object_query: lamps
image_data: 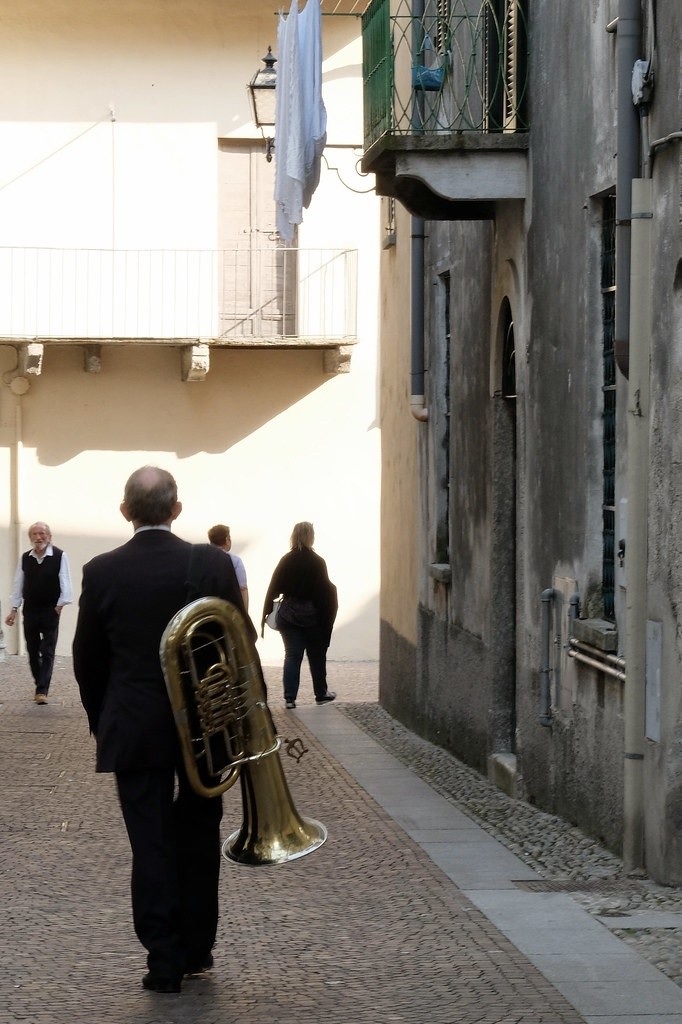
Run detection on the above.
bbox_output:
[248,46,281,165]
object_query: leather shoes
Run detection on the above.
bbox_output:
[189,955,214,975]
[140,973,182,993]
[34,694,48,704]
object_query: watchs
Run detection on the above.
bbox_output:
[11,608,18,614]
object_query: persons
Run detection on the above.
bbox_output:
[207,524,250,618]
[4,520,72,705]
[72,464,266,994]
[259,520,338,709]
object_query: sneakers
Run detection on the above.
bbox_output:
[285,701,295,708]
[316,692,336,704]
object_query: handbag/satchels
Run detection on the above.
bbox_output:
[266,600,283,631]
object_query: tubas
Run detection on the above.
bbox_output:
[158,595,328,868]
